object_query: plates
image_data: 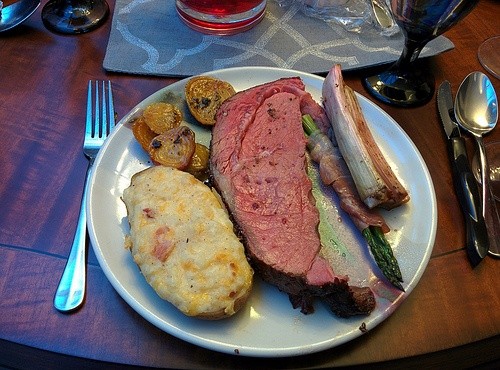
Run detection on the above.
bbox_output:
[87,68,440,357]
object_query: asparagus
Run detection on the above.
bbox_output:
[302,114,406,292]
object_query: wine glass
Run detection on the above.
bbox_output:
[363,0,481,108]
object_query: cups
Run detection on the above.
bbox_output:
[173,1,267,37]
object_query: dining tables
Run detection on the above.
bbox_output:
[0,0,498,369]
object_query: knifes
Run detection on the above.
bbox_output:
[432,81,490,269]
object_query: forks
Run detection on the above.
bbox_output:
[53,79,122,313]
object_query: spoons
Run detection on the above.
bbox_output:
[454,71,500,258]
